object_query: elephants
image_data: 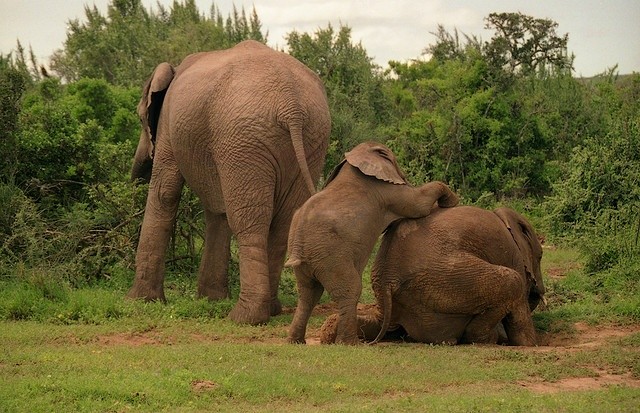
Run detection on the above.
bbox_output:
[282,139,462,346]
[318,204,548,347]
[128,37,333,327]
